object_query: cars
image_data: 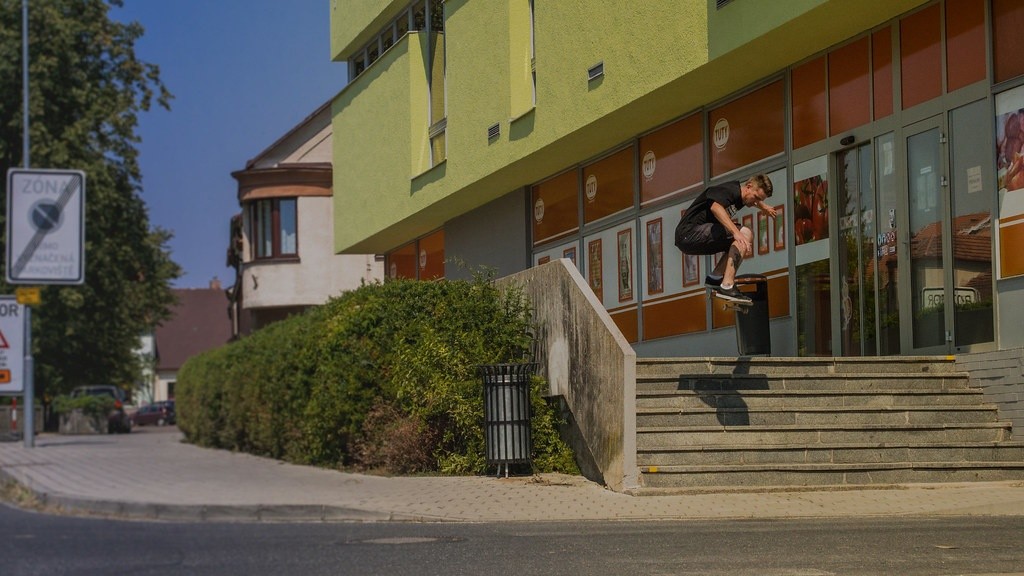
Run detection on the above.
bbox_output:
[69,384,132,434]
[127,400,177,428]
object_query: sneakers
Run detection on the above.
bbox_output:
[704,275,724,289]
[716,284,755,306]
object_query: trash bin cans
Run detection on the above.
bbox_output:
[474,362,540,478]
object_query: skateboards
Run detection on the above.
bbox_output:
[706,284,754,316]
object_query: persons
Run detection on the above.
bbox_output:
[674,173,777,303]
